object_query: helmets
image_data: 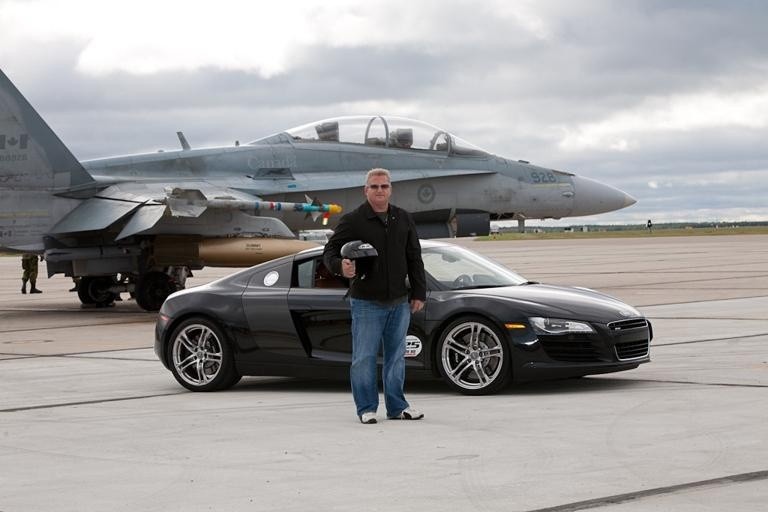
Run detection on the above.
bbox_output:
[340,241,379,278]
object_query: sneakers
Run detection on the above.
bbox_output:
[361,412,377,424]
[397,406,424,420]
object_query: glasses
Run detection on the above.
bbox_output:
[369,184,391,189]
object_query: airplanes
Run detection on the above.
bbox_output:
[1,66,642,315]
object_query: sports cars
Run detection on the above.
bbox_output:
[150,238,653,399]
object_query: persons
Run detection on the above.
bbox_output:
[322,168,427,423]
[21,252,44,293]
[69,278,81,291]
